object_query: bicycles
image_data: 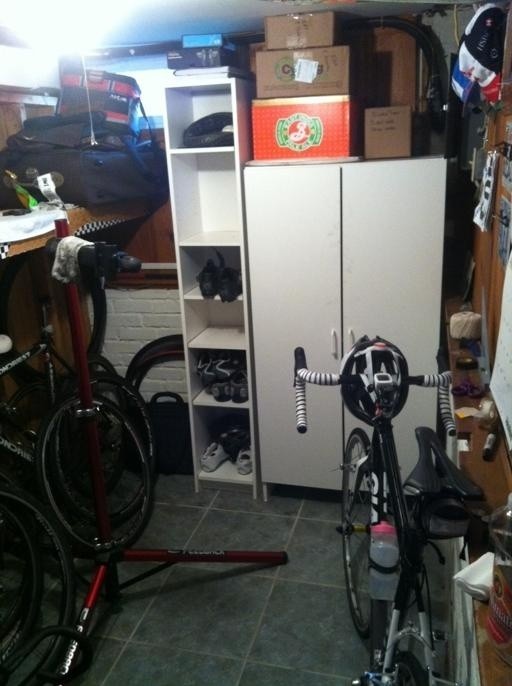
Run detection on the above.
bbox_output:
[293,334,486,686]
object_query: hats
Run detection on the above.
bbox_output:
[450,3,509,118]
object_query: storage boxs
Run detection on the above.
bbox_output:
[264,11,335,51]
[249,41,264,73]
[364,105,411,160]
[167,46,239,69]
[182,34,236,49]
[251,95,358,158]
[256,45,359,98]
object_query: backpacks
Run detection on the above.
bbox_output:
[3,109,167,203]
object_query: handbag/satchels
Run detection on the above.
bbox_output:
[125,390,193,476]
[55,66,144,137]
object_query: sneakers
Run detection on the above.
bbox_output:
[199,429,251,475]
[198,258,241,303]
[197,351,248,403]
[338,334,409,425]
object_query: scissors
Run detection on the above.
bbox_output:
[452,374,484,398]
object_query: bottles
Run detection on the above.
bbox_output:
[368,519,399,603]
[490,491,512,666]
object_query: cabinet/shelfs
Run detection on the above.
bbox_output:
[243,158,448,502]
[163,76,259,501]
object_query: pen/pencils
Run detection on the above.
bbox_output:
[482,422,500,462]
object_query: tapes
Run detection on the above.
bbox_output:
[456,357,478,370]
